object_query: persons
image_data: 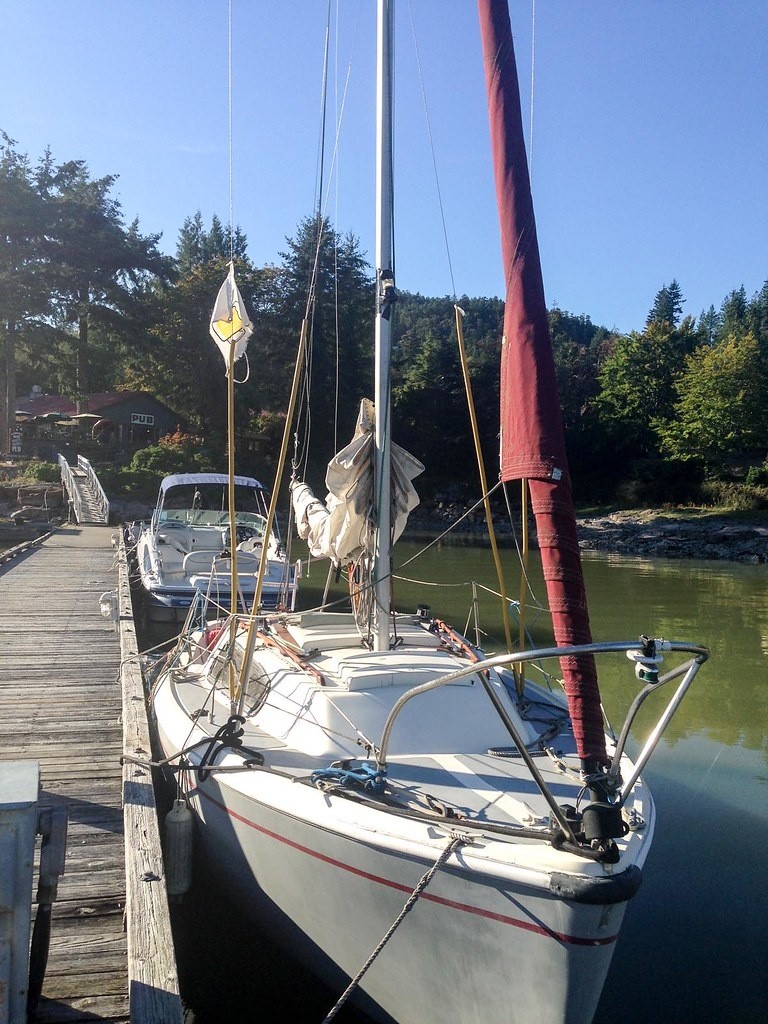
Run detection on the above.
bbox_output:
[194,491,202,509]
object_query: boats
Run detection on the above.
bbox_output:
[136,473,300,622]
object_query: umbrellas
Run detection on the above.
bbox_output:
[15,410,102,440]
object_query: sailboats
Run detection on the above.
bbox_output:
[149,0,709,1024]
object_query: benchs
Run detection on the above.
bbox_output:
[184,550,257,579]
